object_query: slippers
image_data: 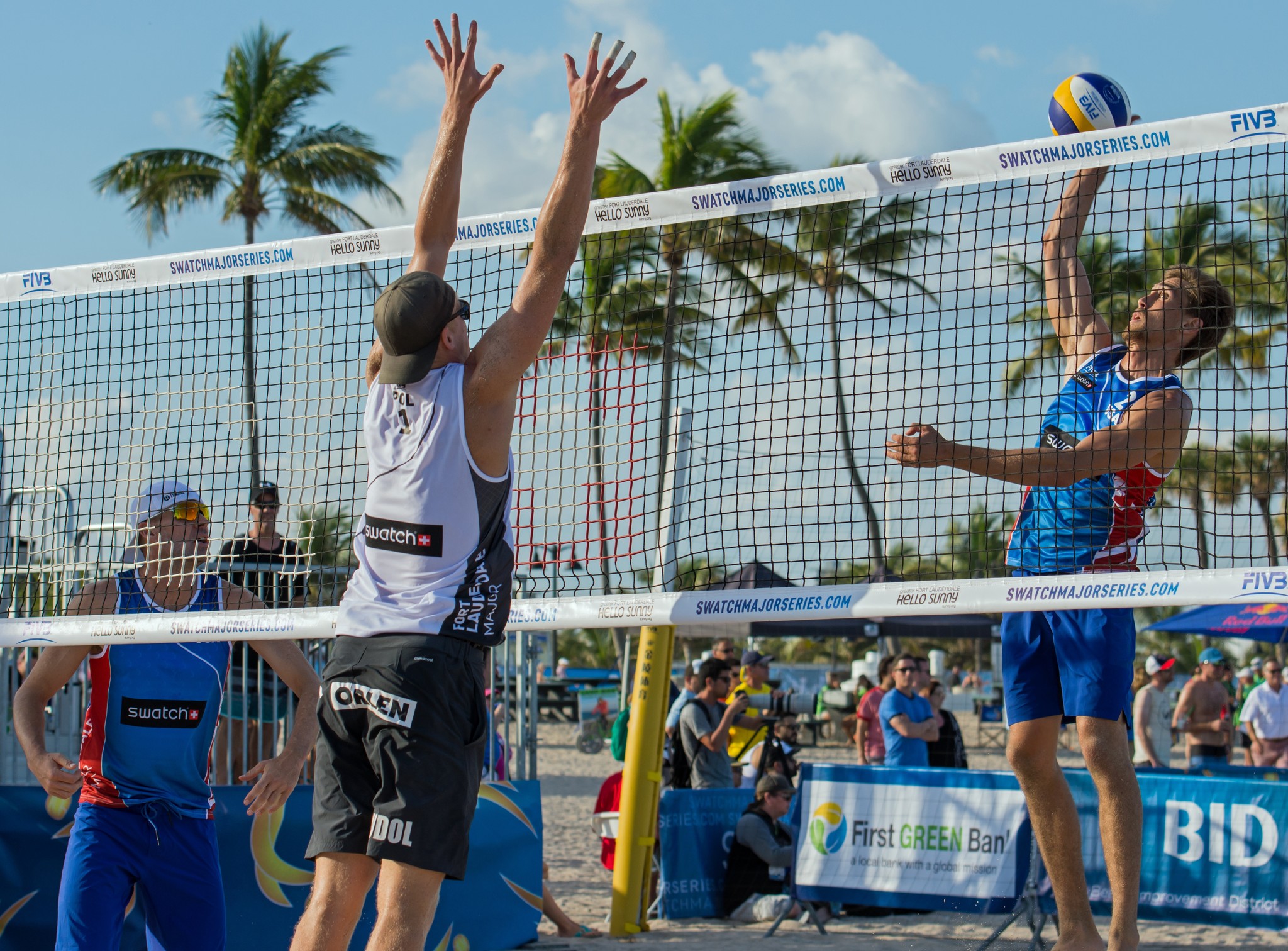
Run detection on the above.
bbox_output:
[574,925,604,938]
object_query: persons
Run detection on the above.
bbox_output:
[649,638,834,927]
[1171,646,1233,767]
[212,482,313,786]
[12,478,323,951]
[816,652,985,770]
[480,645,604,940]
[1131,652,1288,770]
[907,115,1235,951]
[288,12,647,951]
[8,648,41,701]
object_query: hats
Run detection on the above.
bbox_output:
[1199,647,1228,665]
[373,270,457,384]
[485,688,501,697]
[756,775,798,795]
[1250,656,1263,670]
[765,739,801,767]
[1282,666,1288,682]
[740,651,774,666]
[1145,653,1175,677]
[119,480,204,565]
[248,481,280,501]
[1235,666,1253,678]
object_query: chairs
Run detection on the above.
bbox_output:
[785,692,820,747]
[973,695,1006,750]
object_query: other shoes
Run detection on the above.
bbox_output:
[796,909,810,924]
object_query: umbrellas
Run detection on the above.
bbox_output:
[1141,603,1288,646]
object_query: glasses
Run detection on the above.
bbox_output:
[731,672,739,678]
[721,648,734,654]
[715,676,732,683]
[782,796,791,801]
[447,299,472,325]
[1268,669,1283,673]
[778,723,801,730]
[895,666,915,673]
[148,502,211,522]
[253,500,281,509]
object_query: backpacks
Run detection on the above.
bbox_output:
[610,706,632,762]
[669,698,725,790]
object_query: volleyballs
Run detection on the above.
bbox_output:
[1048,71,1132,136]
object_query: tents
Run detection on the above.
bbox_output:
[695,559,1001,642]
